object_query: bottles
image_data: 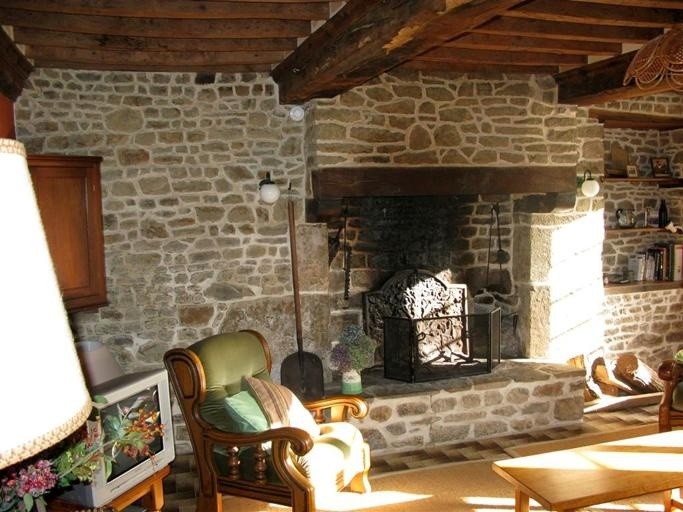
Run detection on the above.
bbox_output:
[657,198,667,228]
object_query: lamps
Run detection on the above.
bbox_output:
[0,136,93,467]
[577,170,599,199]
[254,169,290,206]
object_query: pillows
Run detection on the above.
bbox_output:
[243,375,320,437]
[223,391,270,450]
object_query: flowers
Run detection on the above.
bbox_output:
[328,323,379,374]
[1,400,162,512]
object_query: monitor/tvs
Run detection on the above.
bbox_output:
[55,369,176,509]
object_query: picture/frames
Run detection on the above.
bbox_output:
[625,165,640,178]
[648,157,670,178]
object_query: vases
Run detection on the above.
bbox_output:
[341,370,364,396]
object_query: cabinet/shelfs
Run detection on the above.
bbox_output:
[28,156,110,313]
[604,109,682,294]
[306,167,585,222]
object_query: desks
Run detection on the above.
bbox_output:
[49,463,171,510]
[491,429,683,512]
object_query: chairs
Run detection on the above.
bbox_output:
[656,349,683,432]
[162,329,370,512]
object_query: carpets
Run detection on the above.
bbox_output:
[223,420,683,512]
